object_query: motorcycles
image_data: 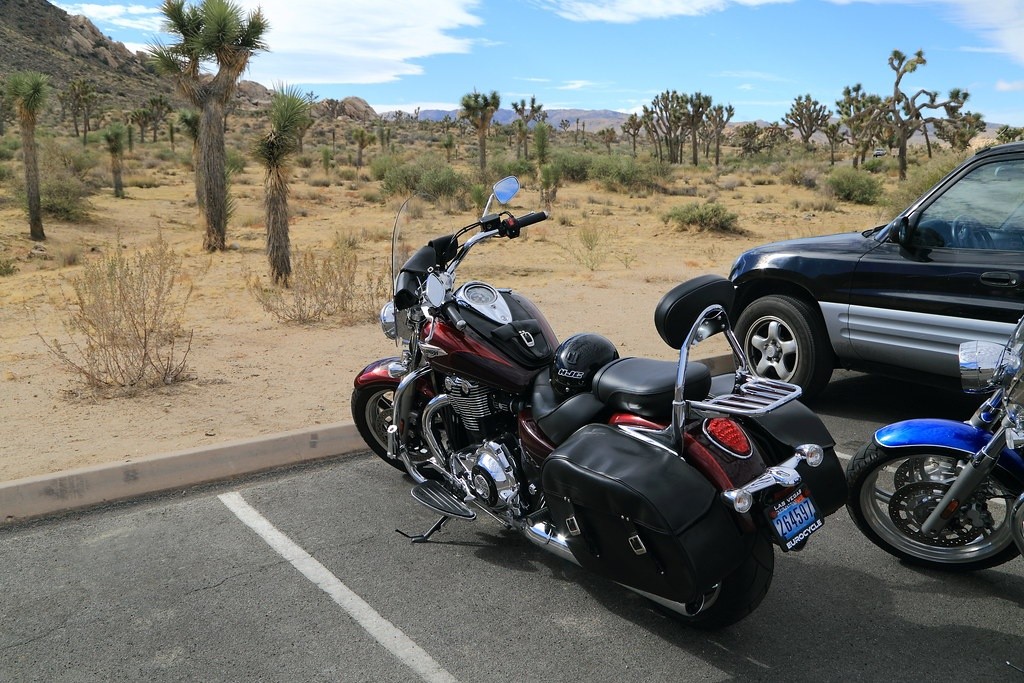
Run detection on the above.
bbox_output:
[352,174,850,636]
[845,302,1024,575]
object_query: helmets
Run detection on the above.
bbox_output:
[550,334,622,403]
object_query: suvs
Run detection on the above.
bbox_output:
[722,138,1022,402]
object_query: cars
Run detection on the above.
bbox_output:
[873,148,887,157]
